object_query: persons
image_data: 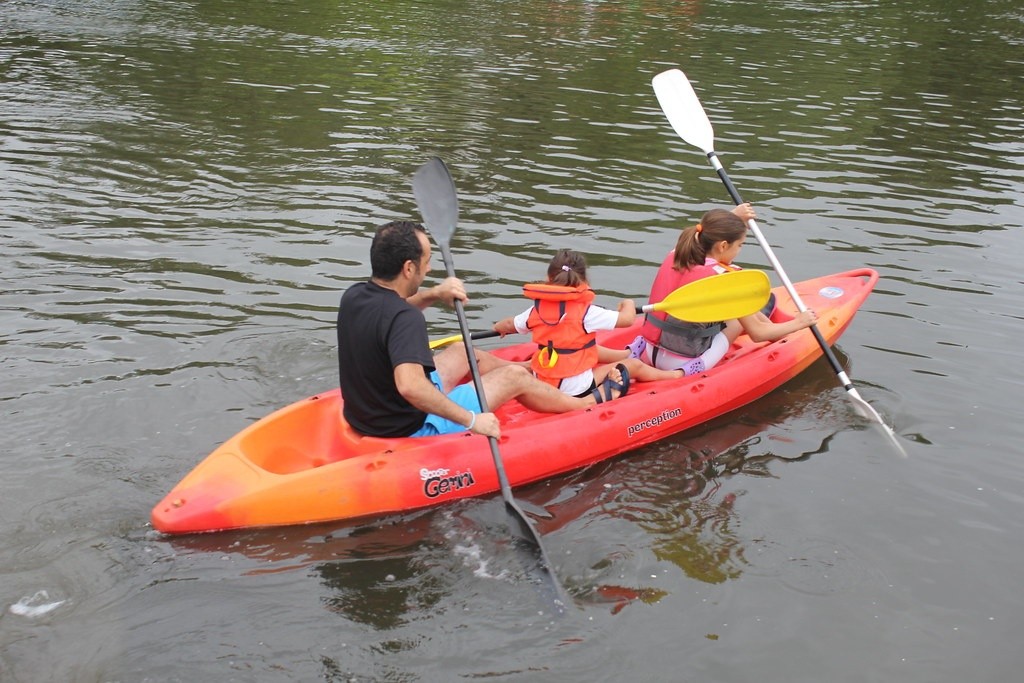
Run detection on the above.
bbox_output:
[631,201,821,386]
[332,215,629,445]
[486,246,706,407]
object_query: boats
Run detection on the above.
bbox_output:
[148,267,880,540]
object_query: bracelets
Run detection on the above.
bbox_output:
[463,409,475,432]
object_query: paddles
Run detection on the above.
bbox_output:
[651,68,899,445]
[423,269,771,350]
[412,154,562,591]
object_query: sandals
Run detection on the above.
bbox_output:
[673,358,705,376]
[589,362,630,404]
[626,335,646,361]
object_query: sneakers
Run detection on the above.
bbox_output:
[759,293,777,319]
